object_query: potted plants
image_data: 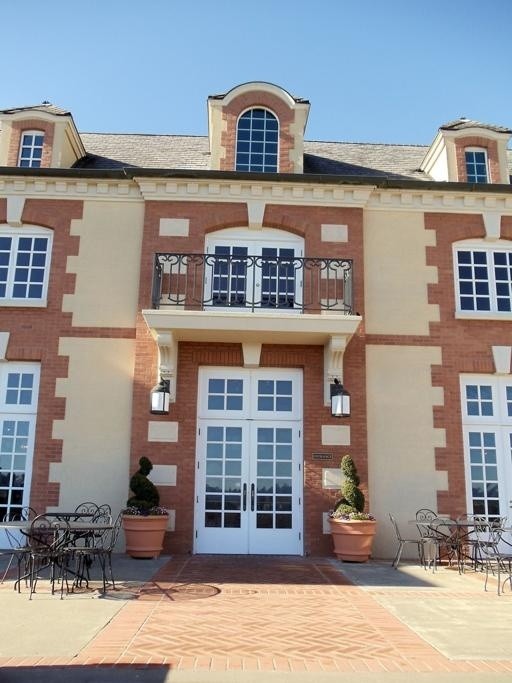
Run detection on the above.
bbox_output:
[324,454,377,563]
[119,455,170,562]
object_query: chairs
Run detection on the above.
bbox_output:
[387,508,512,597]
[0,501,121,600]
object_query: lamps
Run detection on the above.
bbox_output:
[148,375,170,415]
[328,376,351,418]
